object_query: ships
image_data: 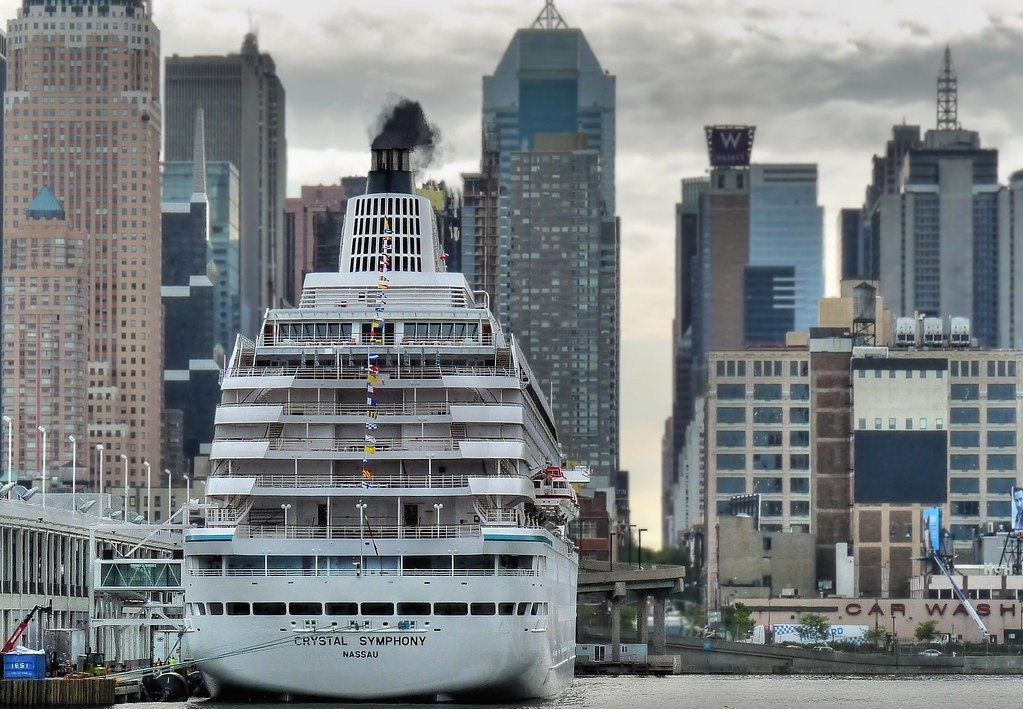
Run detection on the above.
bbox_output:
[177,189,585,701]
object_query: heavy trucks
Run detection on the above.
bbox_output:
[744,622,871,648]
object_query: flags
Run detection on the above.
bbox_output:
[365,422,377,430]
[364,435,376,454]
[367,375,379,384]
[369,365,378,374]
[371,322,384,343]
[369,354,379,361]
[363,469,372,479]
[361,481,373,491]
[378,260,390,289]
[382,216,394,260]
[367,398,378,406]
[367,386,378,395]
[367,411,378,419]
[375,292,387,322]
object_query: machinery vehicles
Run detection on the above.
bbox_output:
[78,651,106,677]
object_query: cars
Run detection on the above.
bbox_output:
[918,649,943,656]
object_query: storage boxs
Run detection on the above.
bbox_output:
[3,653,46,679]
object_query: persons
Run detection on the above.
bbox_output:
[1013,488,1023,529]
[156,658,163,675]
[169,657,175,672]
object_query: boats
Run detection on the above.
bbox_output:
[530,465,580,525]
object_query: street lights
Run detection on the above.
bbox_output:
[639,529,648,570]
[183,475,189,526]
[423,453,434,487]
[302,417,313,444]
[416,417,429,449]
[3,415,12,497]
[144,462,150,525]
[69,434,77,514]
[315,381,324,415]
[120,453,129,523]
[291,453,303,489]
[766,597,773,632]
[433,502,443,540]
[38,425,46,511]
[96,444,105,520]
[355,502,368,540]
[629,524,636,566]
[279,504,291,539]
[164,468,171,525]
[609,531,616,571]
[409,380,421,416]
